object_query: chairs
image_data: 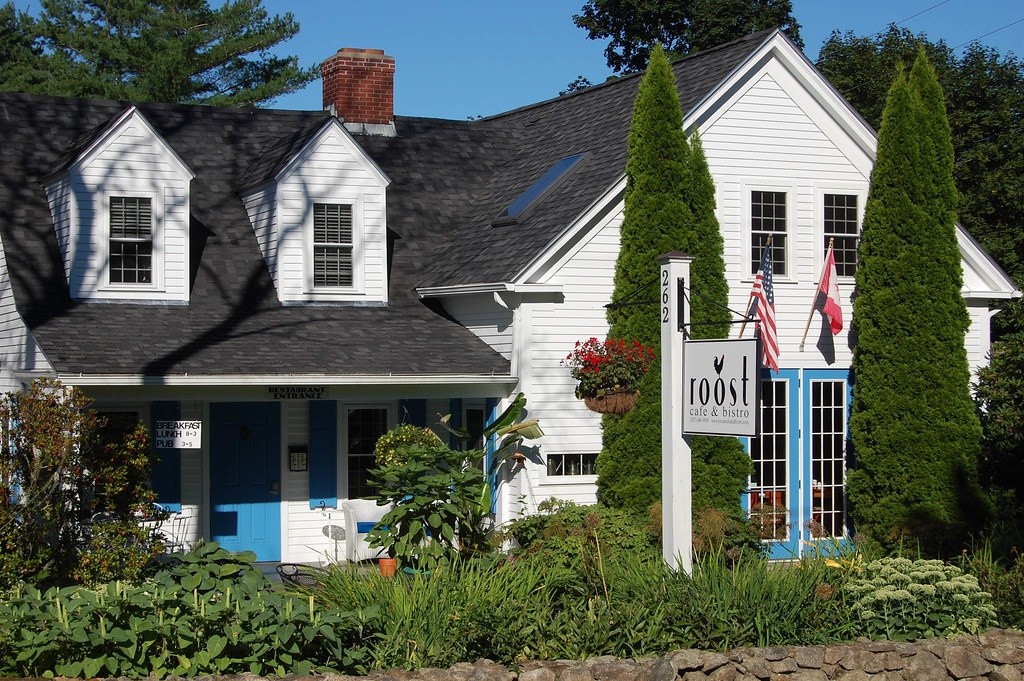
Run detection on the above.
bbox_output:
[163,507,193,552]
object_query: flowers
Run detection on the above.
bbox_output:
[561,336,654,398]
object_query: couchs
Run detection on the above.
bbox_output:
[343,499,400,562]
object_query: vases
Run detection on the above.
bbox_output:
[584,390,639,413]
[379,558,397,577]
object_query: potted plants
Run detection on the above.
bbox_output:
[363,424,485,584]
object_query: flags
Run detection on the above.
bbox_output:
[814,245,843,335]
[746,244,780,375]
